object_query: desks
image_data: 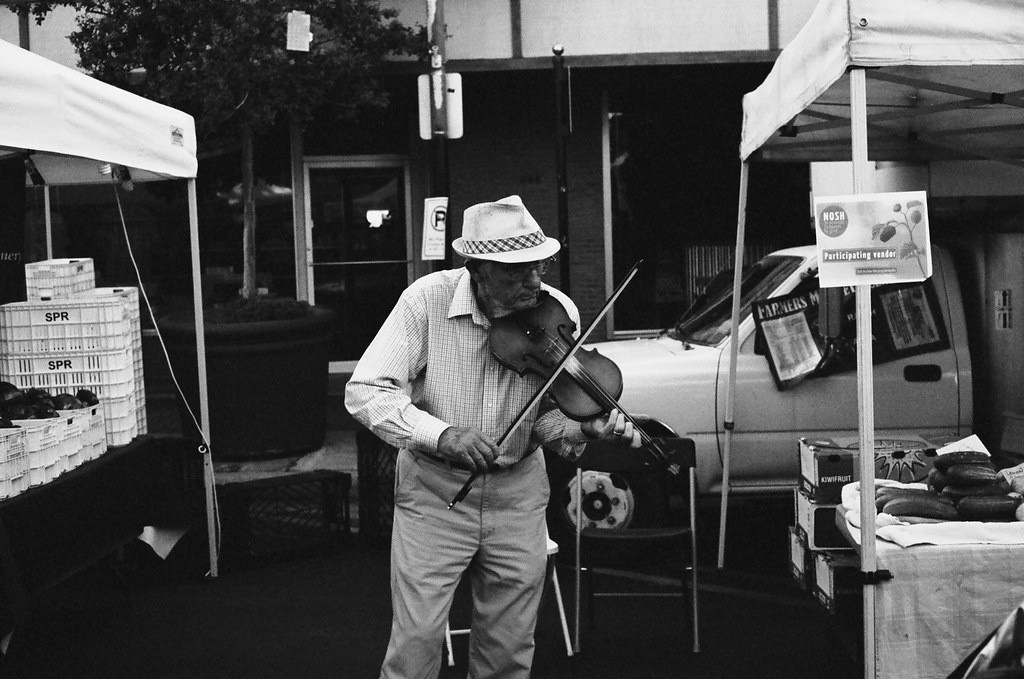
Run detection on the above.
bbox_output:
[0,434,152,601]
[835,504,1024,679]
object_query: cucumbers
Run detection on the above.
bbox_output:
[875,451,1024,522]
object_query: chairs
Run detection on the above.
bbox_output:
[444,526,574,667]
[573,438,700,652]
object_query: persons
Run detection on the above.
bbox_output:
[343,194,641,679]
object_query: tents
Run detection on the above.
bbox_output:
[714,0,1024,679]
[0,37,218,578]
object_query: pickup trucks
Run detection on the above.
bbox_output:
[546,223,1024,552]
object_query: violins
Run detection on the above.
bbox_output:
[488,290,674,471]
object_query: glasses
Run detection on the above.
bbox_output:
[492,256,557,275]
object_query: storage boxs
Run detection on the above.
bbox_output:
[0,258,147,501]
[786,436,938,616]
[219,469,352,575]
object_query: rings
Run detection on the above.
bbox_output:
[613,430,622,436]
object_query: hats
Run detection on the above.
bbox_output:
[451,194,561,262]
